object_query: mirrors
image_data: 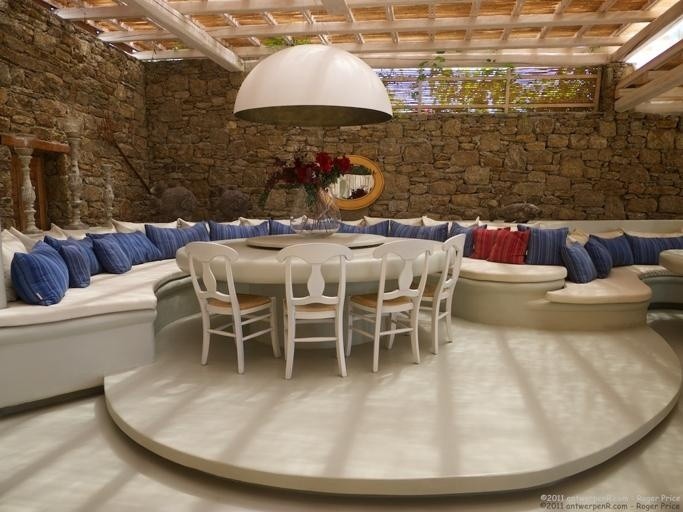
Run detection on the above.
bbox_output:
[320,150,386,211]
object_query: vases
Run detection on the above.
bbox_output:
[288,185,339,234]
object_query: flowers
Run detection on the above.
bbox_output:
[262,149,354,194]
[347,187,370,200]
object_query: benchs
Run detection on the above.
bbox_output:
[0,213,682,418]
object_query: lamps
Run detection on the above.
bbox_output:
[231,43,392,128]
